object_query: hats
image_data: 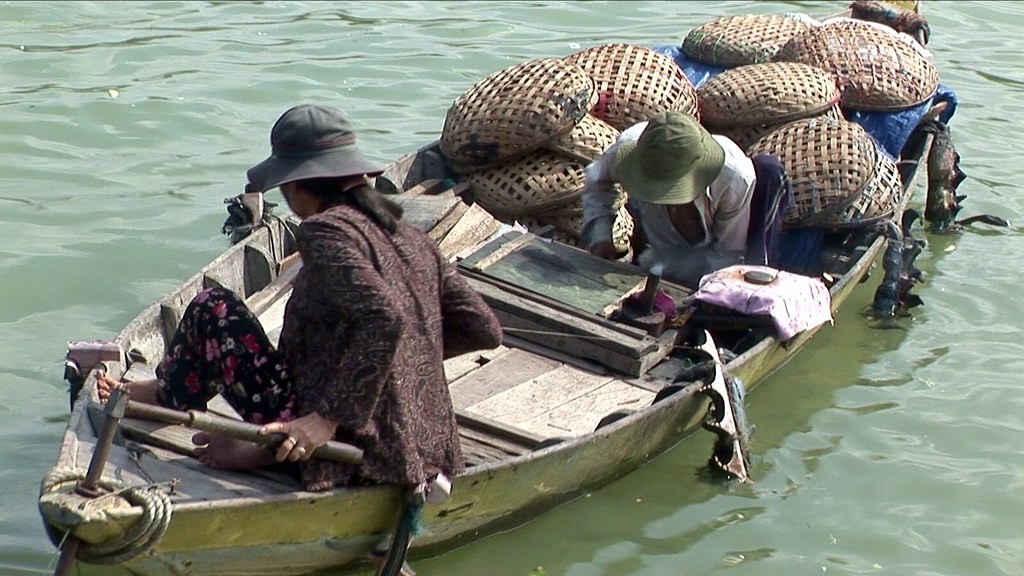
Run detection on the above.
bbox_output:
[615,111,726,205]
[246,104,385,193]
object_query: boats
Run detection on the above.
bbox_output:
[37,0,951,575]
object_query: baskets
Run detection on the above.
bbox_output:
[746,118,878,227]
[812,150,903,228]
[461,148,585,215]
[780,21,938,112]
[696,61,842,128]
[534,196,585,239]
[543,112,620,162]
[708,99,846,152]
[682,14,817,65]
[562,43,701,133]
[440,58,598,166]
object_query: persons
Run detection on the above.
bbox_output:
[580,111,790,291]
[95,105,504,492]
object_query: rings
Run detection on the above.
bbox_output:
[289,437,297,445]
[295,446,307,456]
